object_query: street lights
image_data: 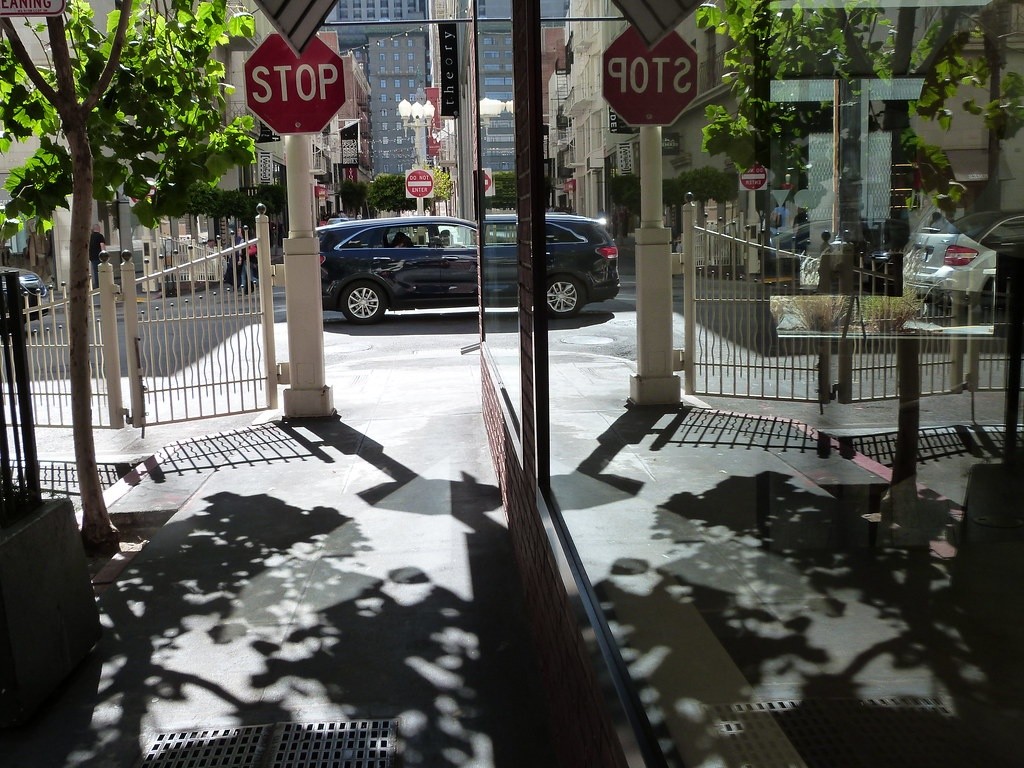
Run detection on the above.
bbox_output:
[400,98,436,246]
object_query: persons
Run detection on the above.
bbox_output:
[323,211,354,221]
[441,230,465,249]
[90,224,107,288]
[206,219,286,292]
[792,209,809,256]
[389,232,412,247]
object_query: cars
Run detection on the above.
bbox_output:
[0,266,49,333]
[899,208,1024,316]
[865,241,905,289]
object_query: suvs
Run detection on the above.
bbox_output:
[317,215,478,324]
[484,214,621,318]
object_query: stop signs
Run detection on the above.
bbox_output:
[483,173,491,193]
[129,181,158,207]
[404,170,432,198]
[738,163,768,190]
[242,31,347,141]
[601,24,702,127]
